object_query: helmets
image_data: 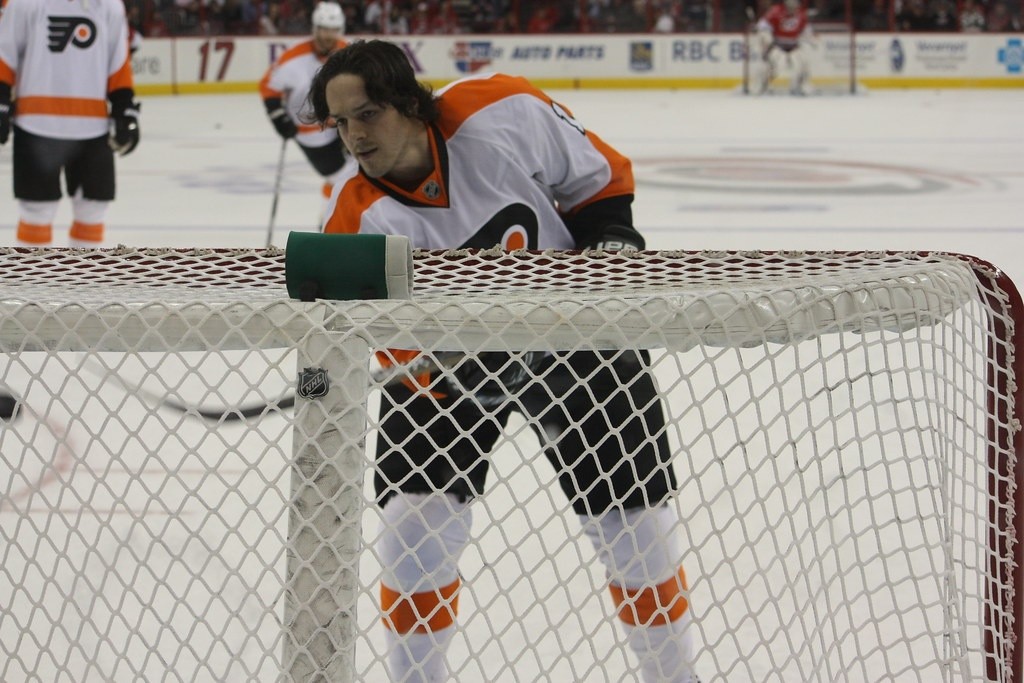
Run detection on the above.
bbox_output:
[311,2,345,55]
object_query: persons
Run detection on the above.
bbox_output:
[755,0,819,95]
[258,0,353,233]
[0,0,142,248]
[124,0,1024,39]
[307,39,704,683]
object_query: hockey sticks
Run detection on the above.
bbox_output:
[77,352,478,423]
[265,137,287,251]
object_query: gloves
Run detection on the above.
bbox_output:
[107,88,141,156]
[0,80,12,143]
[265,98,297,139]
